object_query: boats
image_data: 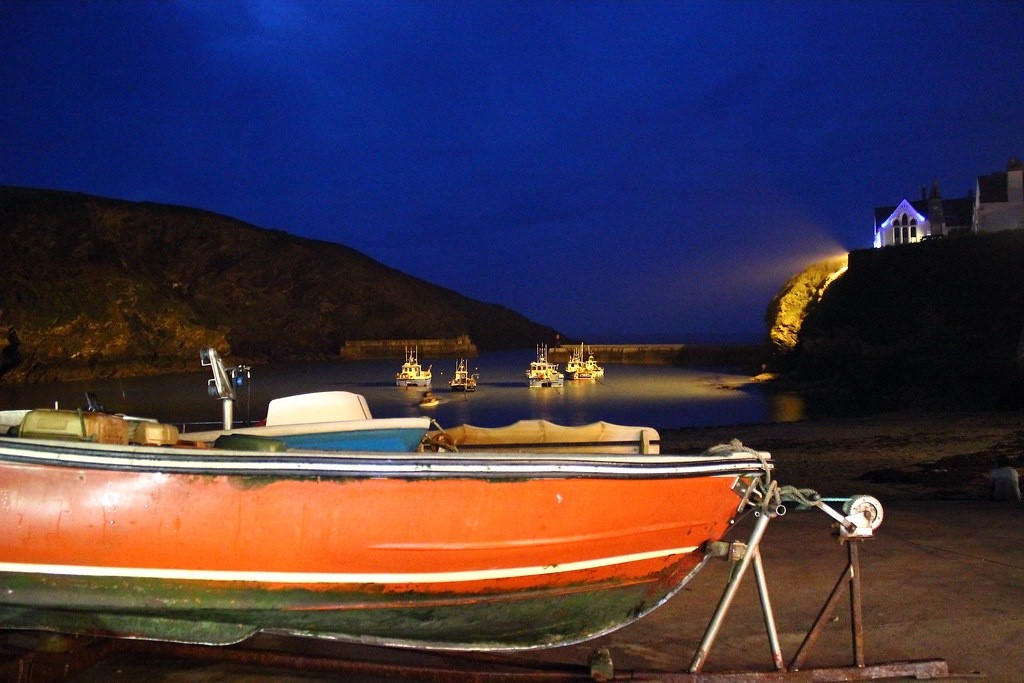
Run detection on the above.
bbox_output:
[417,391,441,407]
[393,345,434,388]
[524,341,565,389]
[563,342,605,380]
[448,357,480,392]
[0,395,773,657]
[119,344,436,452]
